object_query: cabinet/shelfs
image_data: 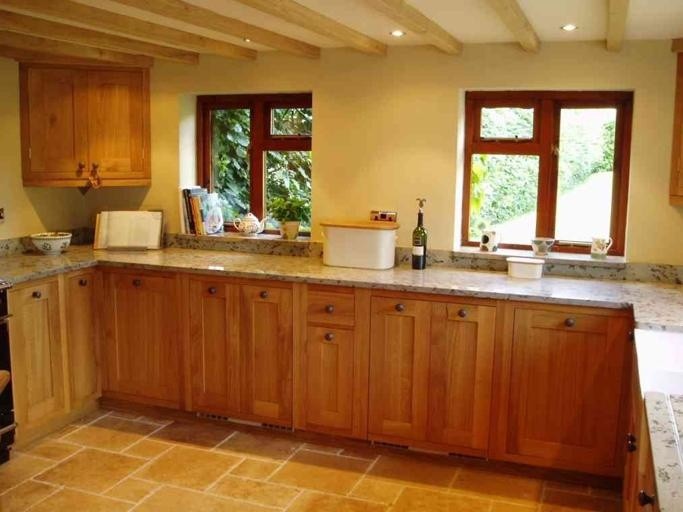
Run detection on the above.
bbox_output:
[669,40,683,208]
[17,61,151,188]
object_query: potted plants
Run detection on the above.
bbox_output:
[267,197,312,239]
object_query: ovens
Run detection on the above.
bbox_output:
[0,278,18,468]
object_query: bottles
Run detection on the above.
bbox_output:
[410,196,432,271]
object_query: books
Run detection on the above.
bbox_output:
[93,210,163,249]
[178,186,223,235]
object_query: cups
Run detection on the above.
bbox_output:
[589,236,614,259]
[479,230,503,252]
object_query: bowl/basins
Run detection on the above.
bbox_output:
[29,230,73,256]
[530,237,555,256]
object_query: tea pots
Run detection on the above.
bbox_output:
[232,207,272,238]
